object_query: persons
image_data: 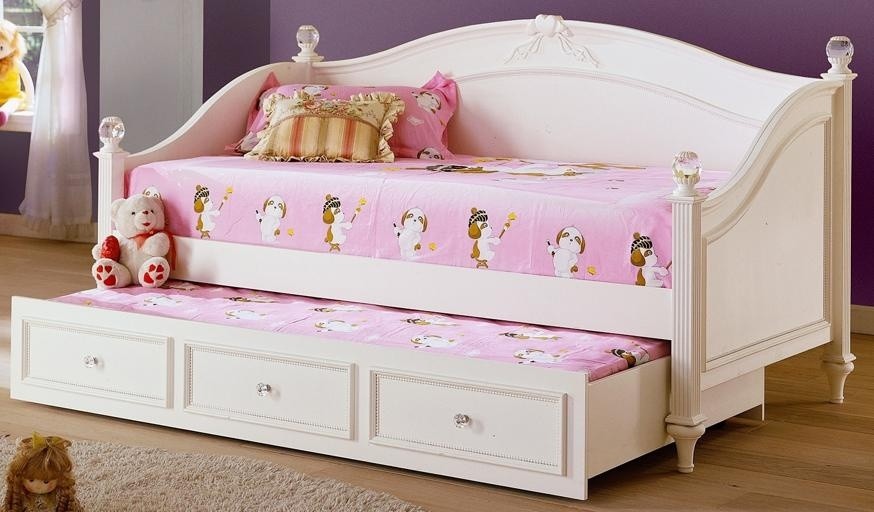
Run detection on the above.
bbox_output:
[0,431,85,512]
[0,16,36,128]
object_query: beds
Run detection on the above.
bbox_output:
[11,14,857,500]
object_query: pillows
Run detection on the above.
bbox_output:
[227,72,459,163]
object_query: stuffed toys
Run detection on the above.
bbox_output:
[90,193,170,290]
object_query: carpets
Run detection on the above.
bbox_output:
[1,435,423,512]
